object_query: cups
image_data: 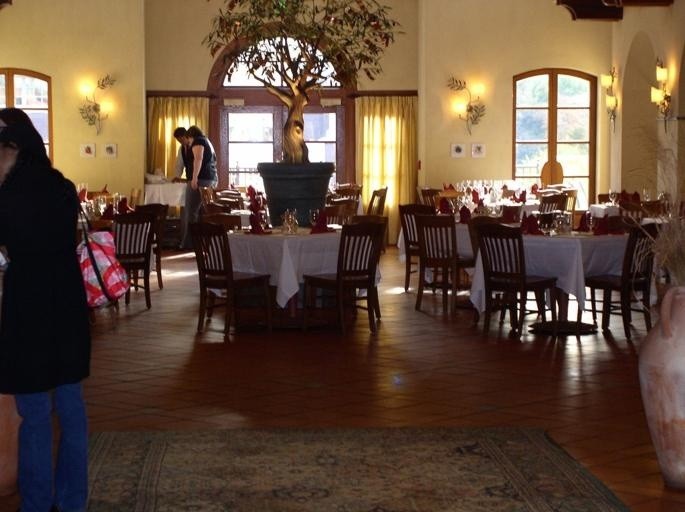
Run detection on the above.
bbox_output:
[308,208,319,225]
[97,192,121,212]
[283,206,298,234]
[255,195,262,208]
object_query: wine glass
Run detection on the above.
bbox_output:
[258,210,267,232]
[608,190,618,206]
[586,211,594,234]
[455,178,530,210]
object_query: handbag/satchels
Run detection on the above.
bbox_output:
[75,230,132,308]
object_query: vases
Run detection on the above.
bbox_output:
[627,284,684,490]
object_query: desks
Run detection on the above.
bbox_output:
[144,182,188,247]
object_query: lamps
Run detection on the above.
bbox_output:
[77,70,117,137]
[646,57,674,133]
[597,62,618,133]
[445,70,490,135]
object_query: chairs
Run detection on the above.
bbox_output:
[74,187,169,328]
[398,183,685,336]
[187,183,390,338]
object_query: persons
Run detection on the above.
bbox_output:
[175,125,217,250]
[171,127,193,183]
[0,105,89,512]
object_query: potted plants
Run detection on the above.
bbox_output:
[201,2,411,227]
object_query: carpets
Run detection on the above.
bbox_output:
[69,418,629,512]
